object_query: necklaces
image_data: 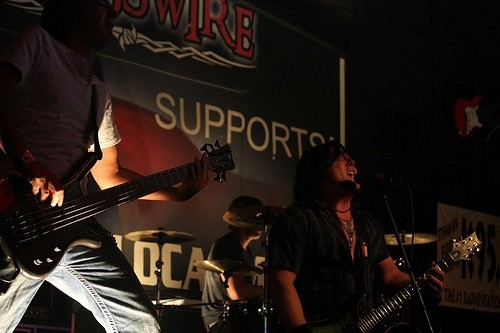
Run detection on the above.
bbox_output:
[339,216,356,248]
[336,207,351,213]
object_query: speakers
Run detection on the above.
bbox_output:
[158,307,208,333]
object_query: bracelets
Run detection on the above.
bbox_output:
[13,149,36,171]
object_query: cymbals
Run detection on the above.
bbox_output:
[384,233,438,246]
[222,205,288,229]
[123,230,196,243]
[188,260,265,277]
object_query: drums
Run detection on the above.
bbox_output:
[223,298,273,319]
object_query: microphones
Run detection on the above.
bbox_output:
[364,170,403,187]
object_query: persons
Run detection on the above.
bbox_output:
[0,0,214,333]
[202,196,264,333]
[265,139,445,333]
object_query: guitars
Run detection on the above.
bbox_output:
[453,94,500,137]
[306,230,482,333]
[0,139,236,276]
